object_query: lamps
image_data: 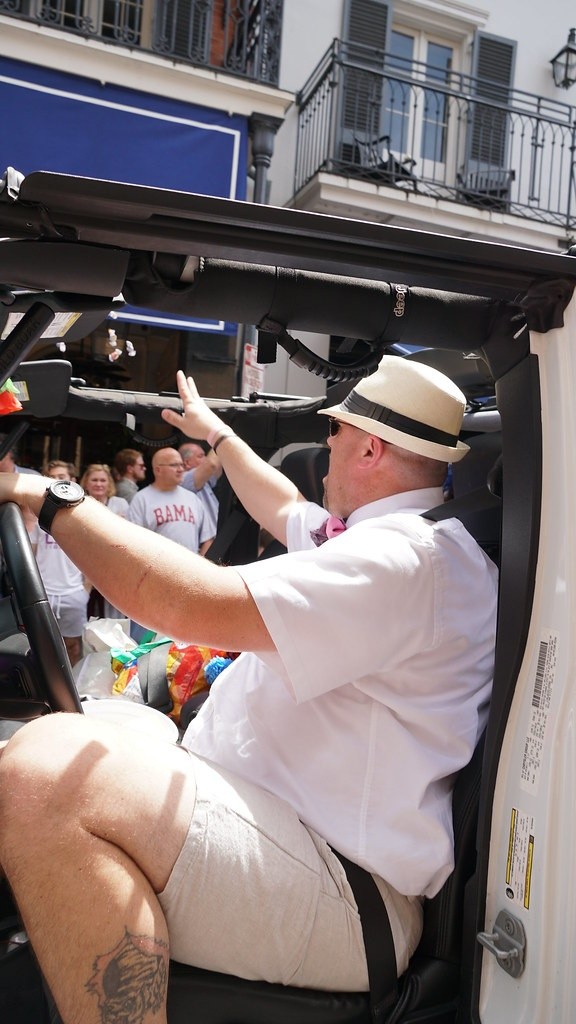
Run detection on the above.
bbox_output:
[549,27,576,90]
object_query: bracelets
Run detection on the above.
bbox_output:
[208,425,237,454]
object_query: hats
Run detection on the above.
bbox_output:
[317,354,471,463]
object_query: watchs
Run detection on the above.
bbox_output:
[38,480,87,535]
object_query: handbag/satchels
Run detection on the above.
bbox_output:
[166,642,227,721]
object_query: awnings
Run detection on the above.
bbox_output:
[394,343,490,405]
[0,64,249,338]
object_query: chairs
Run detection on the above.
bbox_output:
[348,135,419,192]
[456,160,515,212]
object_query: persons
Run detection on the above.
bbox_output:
[0,355,499,1023]
[0,444,222,667]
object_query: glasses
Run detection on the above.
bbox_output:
[158,463,183,468]
[328,417,374,436]
